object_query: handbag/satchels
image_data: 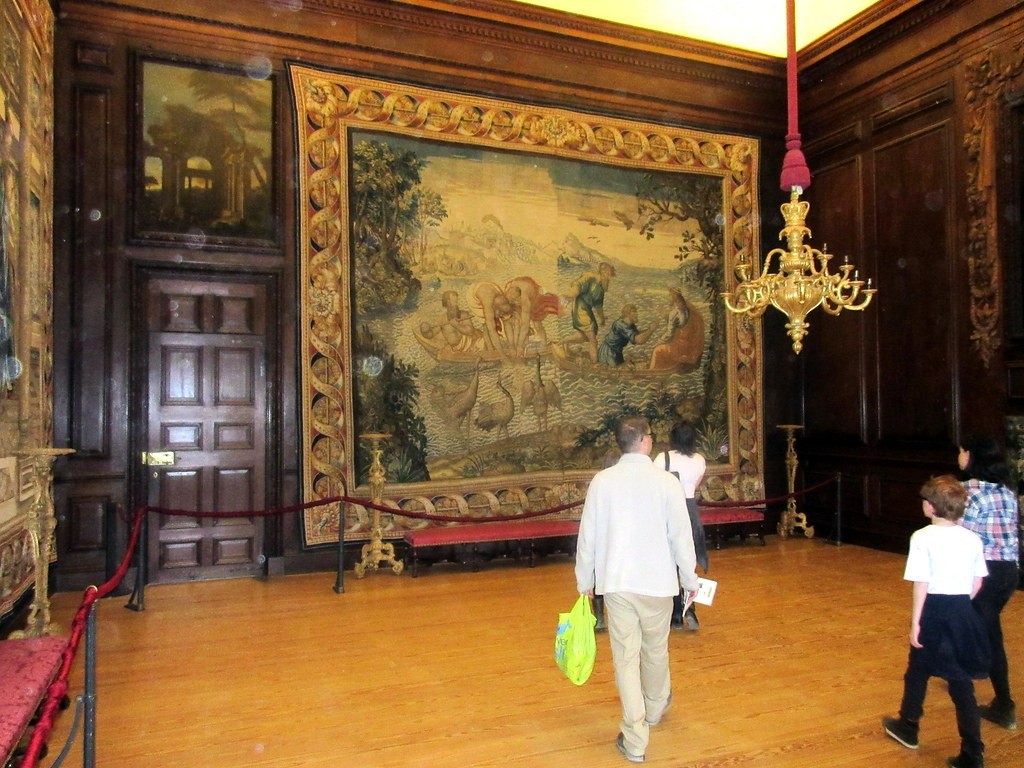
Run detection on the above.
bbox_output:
[665,451,680,481]
[554,593,598,686]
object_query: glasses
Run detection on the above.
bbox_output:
[641,432,654,442]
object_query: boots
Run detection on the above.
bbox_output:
[671,589,683,630]
[683,589,700,630]
[592,598,605,632]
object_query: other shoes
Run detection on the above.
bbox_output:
[946,740,983,768]
[882,715,919,749]
[616,732,645,762]
[977,696,1017,729]
[644,688,672,725]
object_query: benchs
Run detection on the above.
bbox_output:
[403,507,765,578]
[0,636,74,768]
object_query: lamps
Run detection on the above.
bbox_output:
[719,0,878,355]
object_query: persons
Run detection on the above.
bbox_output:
[574,415,699,764]
[953,432,1020,730]
[591,448,622,629]
[881,475,989,768]
[653,421,708,630]
